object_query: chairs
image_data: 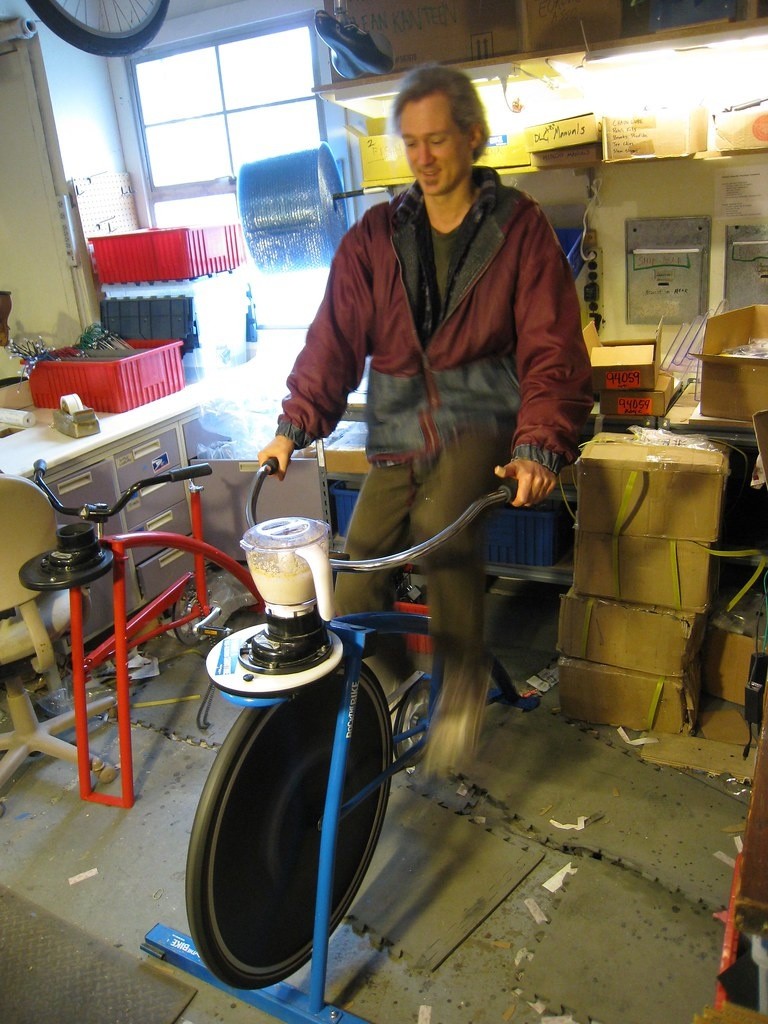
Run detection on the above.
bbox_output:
[0,473,119,818]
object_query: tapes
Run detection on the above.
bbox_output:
[59,392,84,417]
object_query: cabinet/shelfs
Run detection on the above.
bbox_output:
[0,404,203,655]
[316,390,428,592]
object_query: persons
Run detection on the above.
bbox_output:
[259,64,592,779]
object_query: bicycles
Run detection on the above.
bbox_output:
[17,454,266,810]
[178,454,550,1023]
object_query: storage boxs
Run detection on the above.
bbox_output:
[556,590,707,678]
[88,223,248,285]
[482,508,574,567]
[100,271,248,385]
[22,339,187,409]
[330,481,360,537]
[324,433,372,474]
[557,657,700,737]
[394,601,433,655]
[600,372,674,416]
[574,432,731,543]
[582,315,664,393]
[572,528,719,614]
[688,303,768,421]
[701,624,768,706]
